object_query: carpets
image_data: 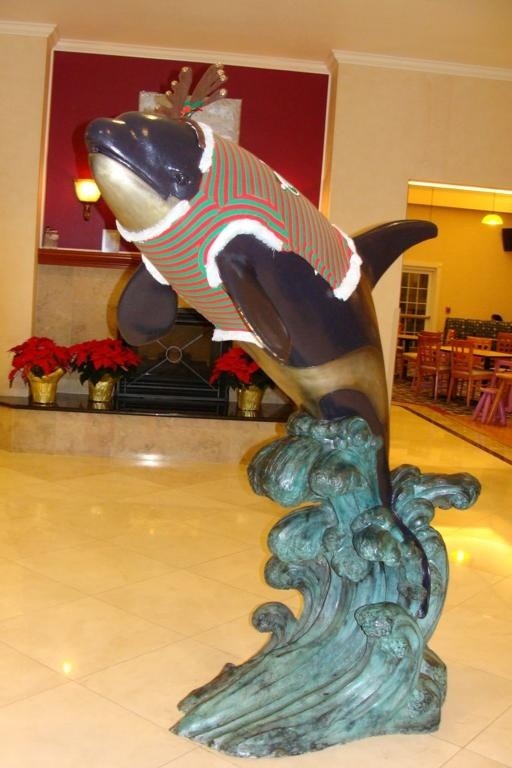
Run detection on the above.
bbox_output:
[392,377,512,465]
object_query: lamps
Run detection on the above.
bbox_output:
[72,177,102,221]
[481,193,503,226]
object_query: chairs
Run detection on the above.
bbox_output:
[395,323,512,426]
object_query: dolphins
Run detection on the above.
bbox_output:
[85,111,438,619]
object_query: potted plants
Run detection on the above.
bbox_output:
[208,346,275,418]
[9,336,74,409]
[67,338,141,410]
